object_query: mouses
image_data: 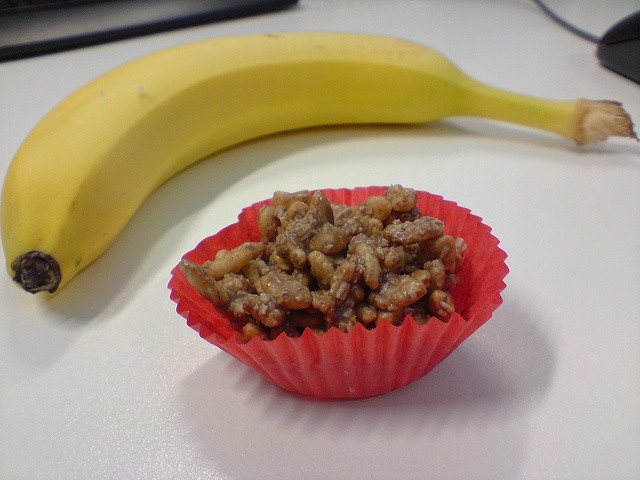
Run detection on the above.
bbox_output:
[596,11,640,85]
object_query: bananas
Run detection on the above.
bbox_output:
[1,31,639,298]
